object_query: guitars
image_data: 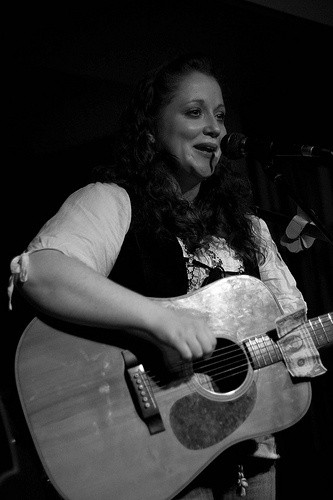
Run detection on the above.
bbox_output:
[16,275,333,500]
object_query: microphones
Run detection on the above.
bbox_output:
[220,132,331,161]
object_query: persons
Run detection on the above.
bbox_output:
[2,56,310,500]
